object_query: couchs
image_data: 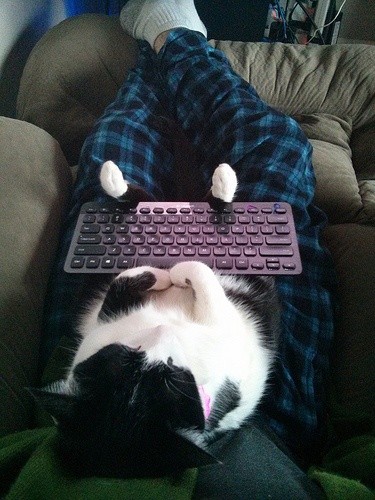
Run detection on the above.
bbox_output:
[0,13,375,499]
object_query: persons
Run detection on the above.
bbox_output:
[25,3,333,500]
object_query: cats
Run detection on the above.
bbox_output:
[51,161,281,475]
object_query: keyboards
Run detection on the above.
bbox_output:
[60,199,303,277]
